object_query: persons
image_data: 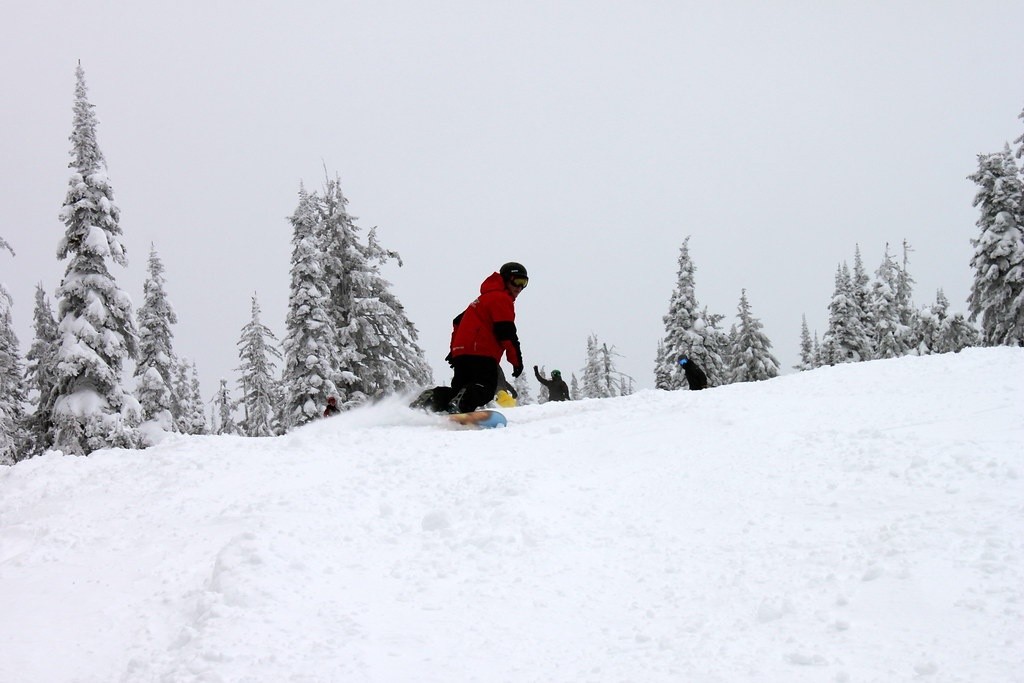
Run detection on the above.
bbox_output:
[411,262,529,420]
[533,366,570,402]
[495,364,518,407]
[678,355,707,391]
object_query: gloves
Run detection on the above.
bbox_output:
[512,363,523,378]
[445,353,455,369]
[512,391,517,399]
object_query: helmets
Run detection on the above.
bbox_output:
[500,262,527,277]
[677,354,686,364]
[551,370,561,378]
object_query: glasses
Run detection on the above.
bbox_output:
[512,277,528,288]
[680,359,687,365]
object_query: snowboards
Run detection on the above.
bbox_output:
[435,410,508,431]
